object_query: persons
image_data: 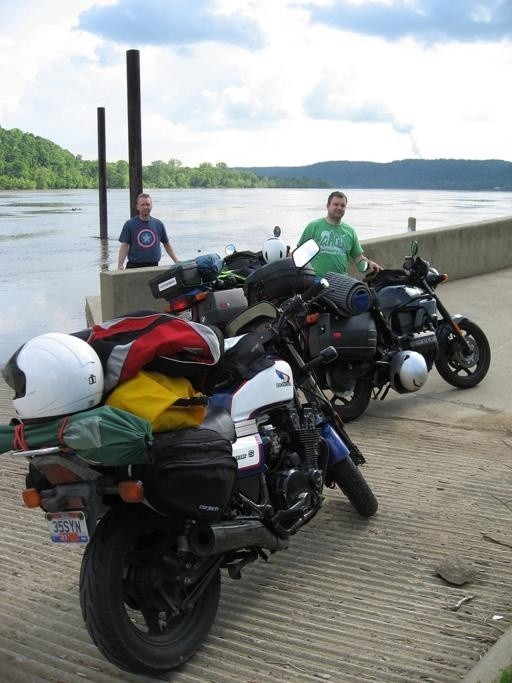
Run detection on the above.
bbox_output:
[296,190,383,389]
[116,192,182,269]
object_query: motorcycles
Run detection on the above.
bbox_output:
[149,218,491,423]
[10,237,377,674]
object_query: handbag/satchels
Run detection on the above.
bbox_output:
[138,428,238,520]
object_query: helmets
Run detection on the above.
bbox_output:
[261,237,287,263]
[392,350,427,393]
[2,333,105,418]
